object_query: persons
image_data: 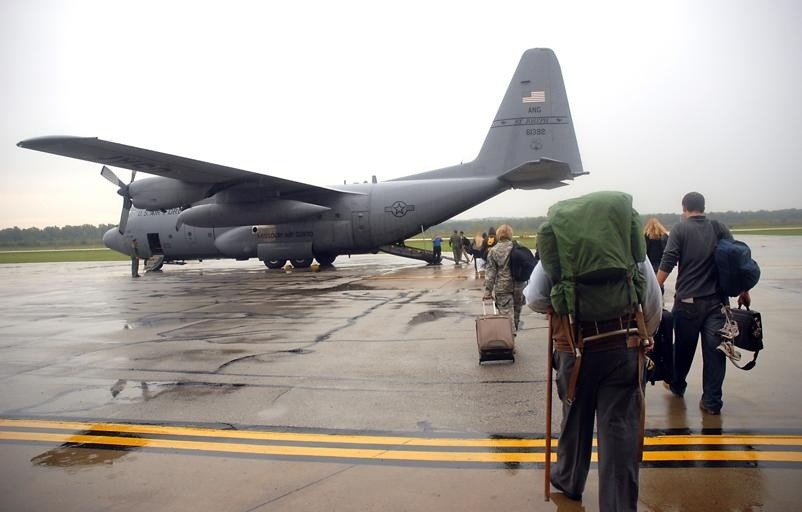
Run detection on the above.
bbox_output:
[449,227,496,271]
[657,194,750,414]
[483,224,526,337]
[132,239,141,278]
[433,236,442,265]
[522,255,662,512]
[644,218,669,304]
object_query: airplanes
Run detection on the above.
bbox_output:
[16,48,590,270]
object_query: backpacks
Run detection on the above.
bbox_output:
[537,191,649,344]
[711,220,760,297]
[510,240,535,282]
[644,309,674,380]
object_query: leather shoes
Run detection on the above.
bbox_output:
[548,462,585,501]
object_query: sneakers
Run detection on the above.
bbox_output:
[698,396,723,415]
[716,341,741,361]
[663,381,687,398]
[716,320,740,340]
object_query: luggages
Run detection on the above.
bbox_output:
[475,297,515,365]
